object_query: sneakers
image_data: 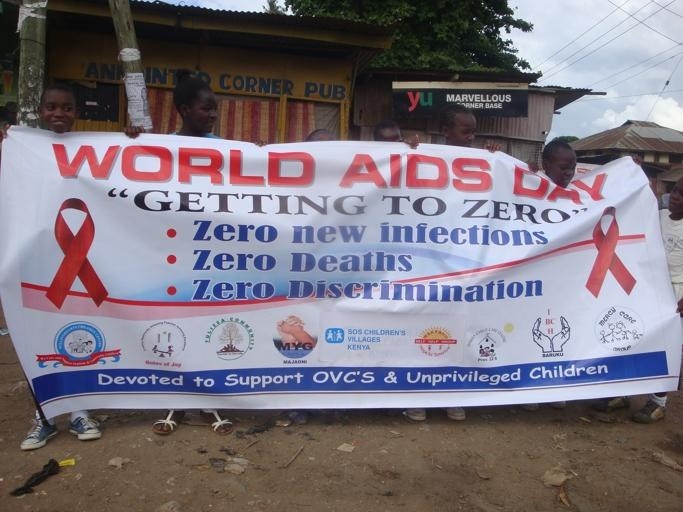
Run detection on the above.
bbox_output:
[20,416,58,451]
[447,407,466,421]
[595,397,629,410]
[631,398,665,424]
[408,407,426,422]
[521,401,567,411]
[68,417,103,440]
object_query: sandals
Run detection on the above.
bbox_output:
[152,409,185,435]
[204,410,233,435]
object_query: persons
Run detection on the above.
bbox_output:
[406,104,501,420]
[1,80,102,450]
[374,122,404,143]
[124,78,265,436]
[290,129,337,425]
[521,137,577,409]
[593,152,682,423]
